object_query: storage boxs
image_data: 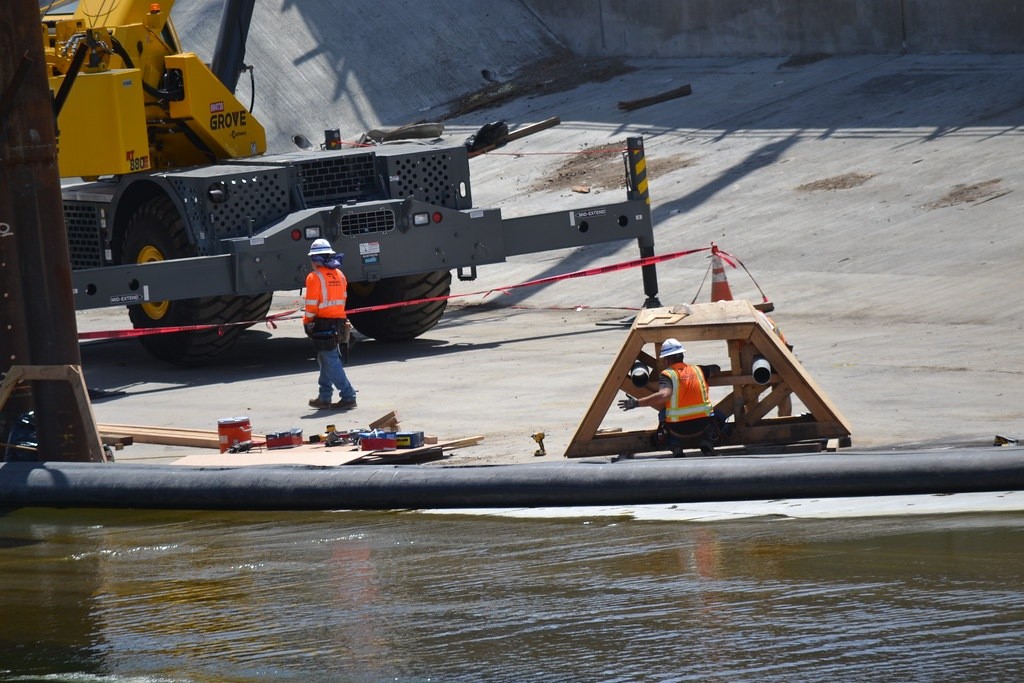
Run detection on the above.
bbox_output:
[358,433,396,439]
[267,436,302,449]
[369,410,408,433]
[396,431,424,448]
[361,439,396,452]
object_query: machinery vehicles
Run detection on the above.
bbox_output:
[39,0,663,368]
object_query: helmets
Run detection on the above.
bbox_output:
[659,338,687,358]
[307,239,336,256]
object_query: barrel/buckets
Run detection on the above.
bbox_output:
[217,417,255,455]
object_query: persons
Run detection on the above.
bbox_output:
[303,238,357,410]
[617,338,727,458]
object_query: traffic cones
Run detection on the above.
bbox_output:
[711,244,733,301]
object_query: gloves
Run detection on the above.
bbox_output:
[618,393,639,411]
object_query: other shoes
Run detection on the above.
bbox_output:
[673,447,686,457]
[699,439,719,456]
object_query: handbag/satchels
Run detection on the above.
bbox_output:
[313,334,337,352]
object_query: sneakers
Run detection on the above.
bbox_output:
[335,398,357,409]
[309,399,330,408]
